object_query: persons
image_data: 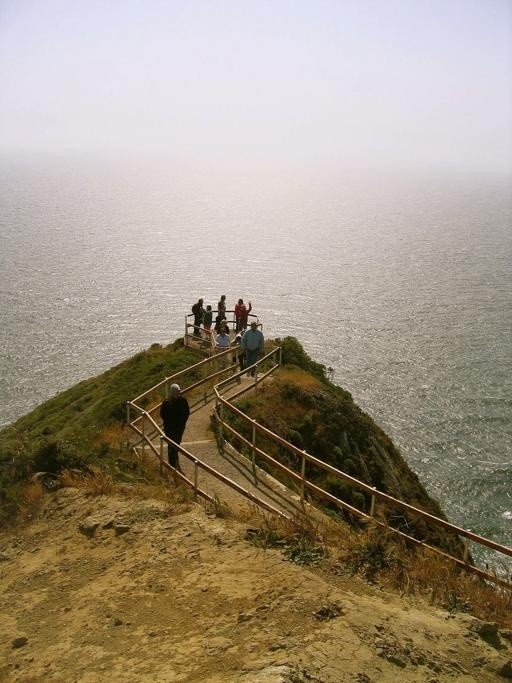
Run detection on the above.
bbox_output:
[192,295,265,377]
[160,383,190,477]
